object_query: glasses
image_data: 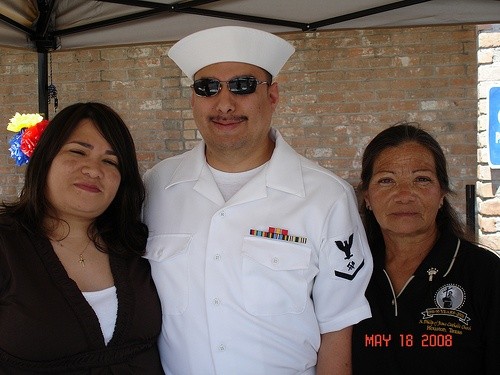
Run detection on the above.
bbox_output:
[190,75,270,97]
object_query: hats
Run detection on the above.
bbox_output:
[167,26,295,84]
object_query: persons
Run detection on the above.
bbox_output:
[142,25,373,375]
[0,102,164,375]
[350,124,500,374]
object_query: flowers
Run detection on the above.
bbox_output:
[6,111,50,165]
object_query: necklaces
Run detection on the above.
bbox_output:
[45,225,97,266]
